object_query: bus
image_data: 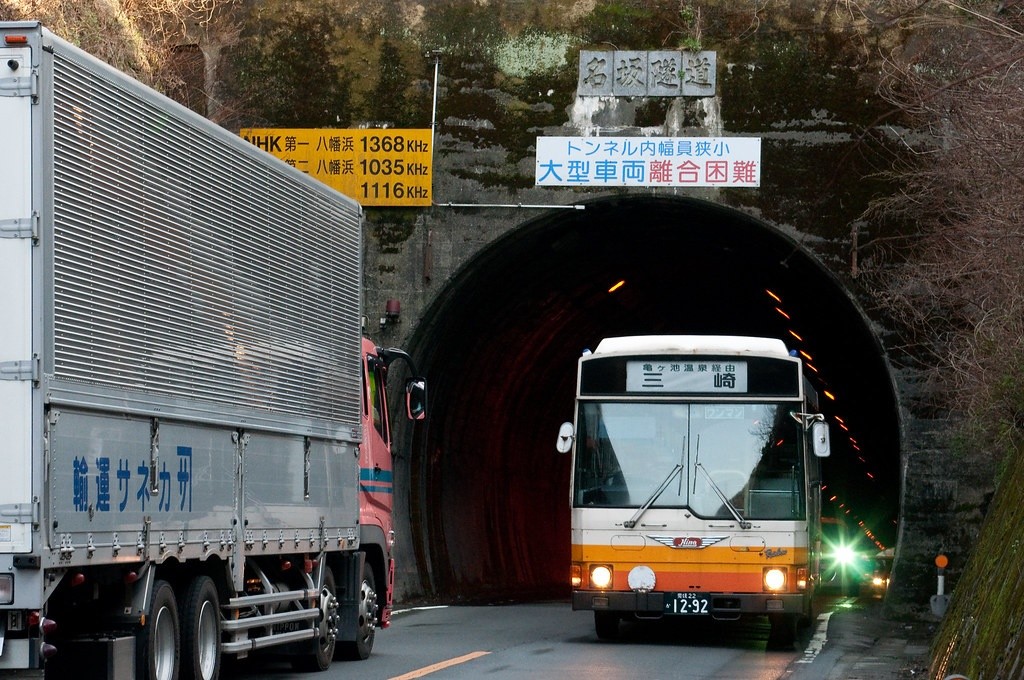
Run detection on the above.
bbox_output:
[555,333,863,647]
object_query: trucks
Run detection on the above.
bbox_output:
[0,21,427,680]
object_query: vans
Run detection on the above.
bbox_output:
[871,548,896,596]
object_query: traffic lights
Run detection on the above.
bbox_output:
[935,553,955,571]
